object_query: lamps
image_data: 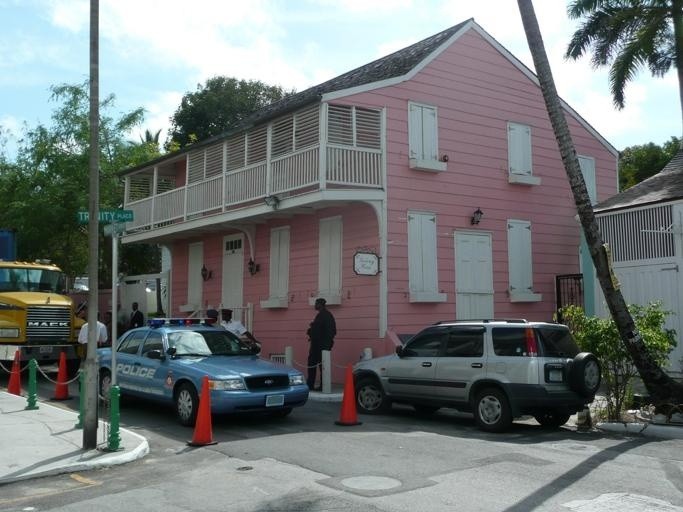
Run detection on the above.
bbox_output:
[471,207,484,224]
[201,263,211,281]
[249,258,259,275]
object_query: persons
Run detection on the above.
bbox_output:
[305,298,336,391]
[219,307,262,353]
[74,300,145,365]
[206,307,224,330]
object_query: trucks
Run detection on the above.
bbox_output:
[0,259,88,376]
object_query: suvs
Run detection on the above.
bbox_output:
[350,318,601,432]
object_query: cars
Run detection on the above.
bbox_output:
[76,319,309,427]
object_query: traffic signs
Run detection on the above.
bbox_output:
[75,210,133,224]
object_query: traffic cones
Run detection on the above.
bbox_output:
[7,350,22,395]
[185,376,217,446]
[335,364,363,426]
[49,354,75,401]
